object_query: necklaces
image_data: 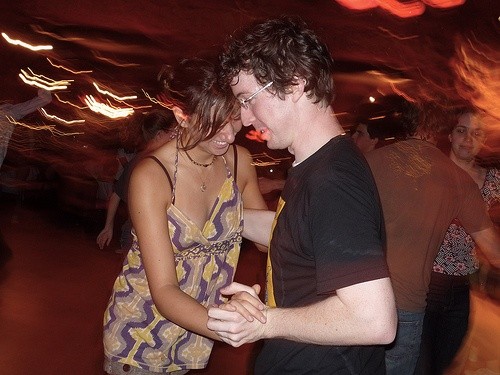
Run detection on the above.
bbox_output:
[178,136,219,192]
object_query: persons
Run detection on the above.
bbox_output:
[1,16,500,375]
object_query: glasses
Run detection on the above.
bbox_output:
[236,81,275,110]
